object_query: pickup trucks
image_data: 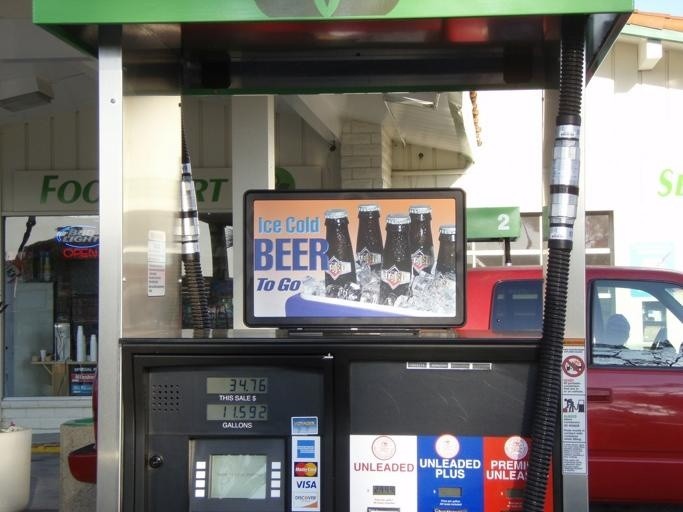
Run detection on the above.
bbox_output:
[66,264,681,510]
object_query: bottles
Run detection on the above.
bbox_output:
[324,205,456,302]
[35,250,51,283]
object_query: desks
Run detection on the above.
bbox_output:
[30,359,97,395]
[0,426,33,511]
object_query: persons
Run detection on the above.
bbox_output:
[603,312,634,349]
